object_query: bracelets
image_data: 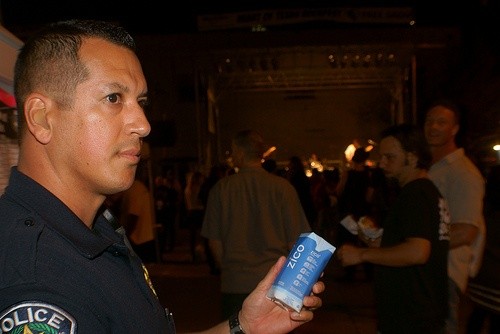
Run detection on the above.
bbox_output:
[228,310,250,334]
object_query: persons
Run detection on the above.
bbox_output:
[337,123,452,334]
[182,155,209,263]
[201,129,315,322]
[309,166,330,232]
[285,152,311,227]
[341,145,378,290]
[119,142,161,299]
[1,16,326,334]
[419,96,486,329]
